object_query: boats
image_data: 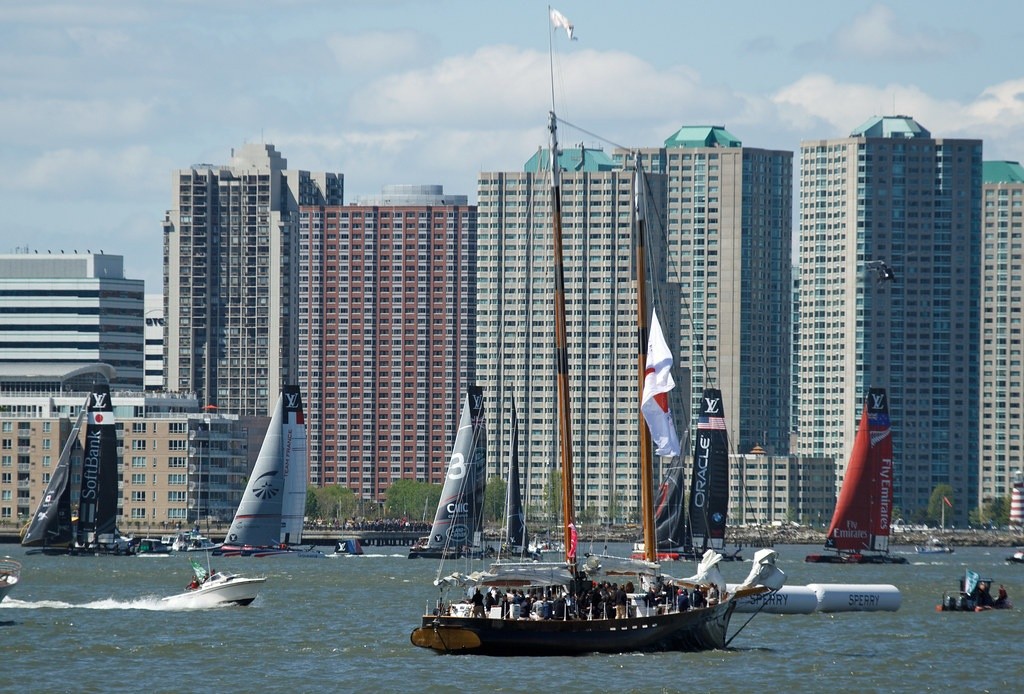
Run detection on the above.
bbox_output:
[0,559,23,604]
[134,529,186,555]
[937,567,1013,611]
[1005,551,1024,566]
[531,529,566,554]
[915,541,954,554]
[324,538,363,558]
[160,543,267,607]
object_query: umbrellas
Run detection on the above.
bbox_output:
[202,405,218,409]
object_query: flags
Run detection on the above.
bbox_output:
[550,7,578,40]
[640,307,681,458]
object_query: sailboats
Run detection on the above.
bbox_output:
[212,385,318,560]
[20,383,135,556]
[406,385,491,559]
[804,387,912,565]
[629,387,746,562]
[185,442,224,552]
[495,382,544,559]
[410,108,793,657]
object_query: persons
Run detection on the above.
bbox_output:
[999,585,1007,599]
[488,545,495,553]
[185,569,215,589]
[467,576,729,620]
